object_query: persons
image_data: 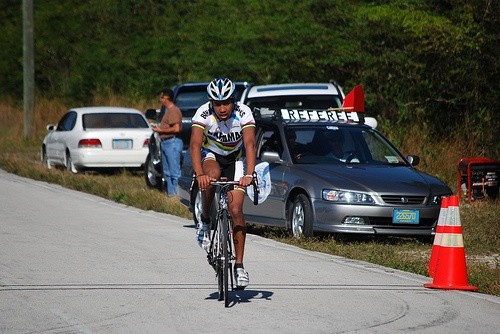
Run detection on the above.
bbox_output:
[151,89,183,199]
[191,77,256,287]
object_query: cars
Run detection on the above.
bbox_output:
[144,81,454,242]
[40,106,155,174]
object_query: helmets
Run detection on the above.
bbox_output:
[205,77,236,102]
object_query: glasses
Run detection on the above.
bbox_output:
[288,135,297,140]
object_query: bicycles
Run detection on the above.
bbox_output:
[196,172,261,308]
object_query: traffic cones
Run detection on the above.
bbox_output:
[424,195,479,292]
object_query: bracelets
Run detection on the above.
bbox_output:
[244,174,254,178]
[197,174,206,177]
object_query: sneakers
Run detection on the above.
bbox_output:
[234,267,249,287]
[195,216,211,249]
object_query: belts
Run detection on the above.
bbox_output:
[161,136,174,142]
